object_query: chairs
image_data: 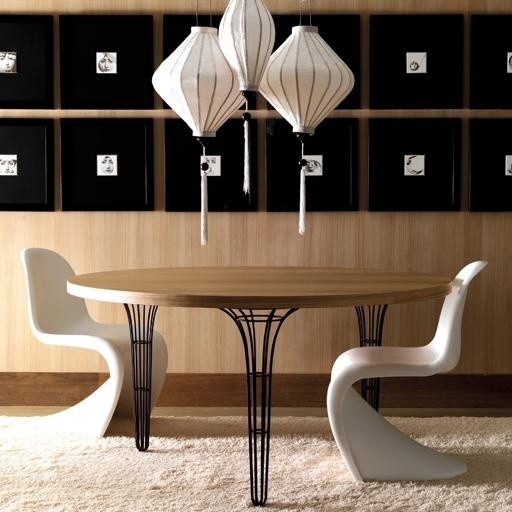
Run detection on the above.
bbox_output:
[326,259,491,482]
[18,246,171,442]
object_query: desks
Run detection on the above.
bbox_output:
[64,268,454,507]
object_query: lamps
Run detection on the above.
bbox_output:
[153,1,355,246]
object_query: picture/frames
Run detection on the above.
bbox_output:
[2,2,511,216]
